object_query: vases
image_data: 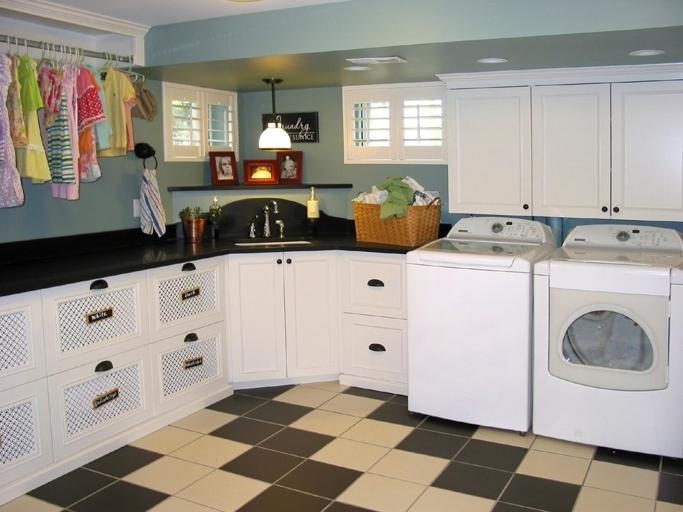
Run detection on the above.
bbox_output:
[208,221,220,238]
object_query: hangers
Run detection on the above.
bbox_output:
[5,33,145,86]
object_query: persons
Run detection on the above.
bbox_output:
[216,157,233,180]
[280,155,296,178]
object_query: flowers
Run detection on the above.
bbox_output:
[208,195,224,220]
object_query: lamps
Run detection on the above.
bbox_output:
[256,76,292,152]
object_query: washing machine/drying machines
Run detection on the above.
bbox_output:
[532,223,683,460]
[404,216,556,437]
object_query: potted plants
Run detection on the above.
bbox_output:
[177,205,207,243]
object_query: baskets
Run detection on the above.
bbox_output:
[350,185,443,249]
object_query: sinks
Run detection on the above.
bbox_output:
[234,239,312,246]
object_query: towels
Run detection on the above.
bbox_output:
[137,169,167,238]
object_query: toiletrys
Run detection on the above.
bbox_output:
[208,195,225,217]
[305,186,320,219]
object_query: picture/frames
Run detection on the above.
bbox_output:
[277,150,303,184]
[207,149,238,187]
[242,158,279,185]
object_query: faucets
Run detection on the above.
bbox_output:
[263,199,279,237]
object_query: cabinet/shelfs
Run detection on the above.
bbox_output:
[447,86,533,217]
[44,270,155,476]
[229,251,340,389]
[339,253,408,396]
[535,78,683,224]
[148,255,228,428]
[1,289,53,486]
